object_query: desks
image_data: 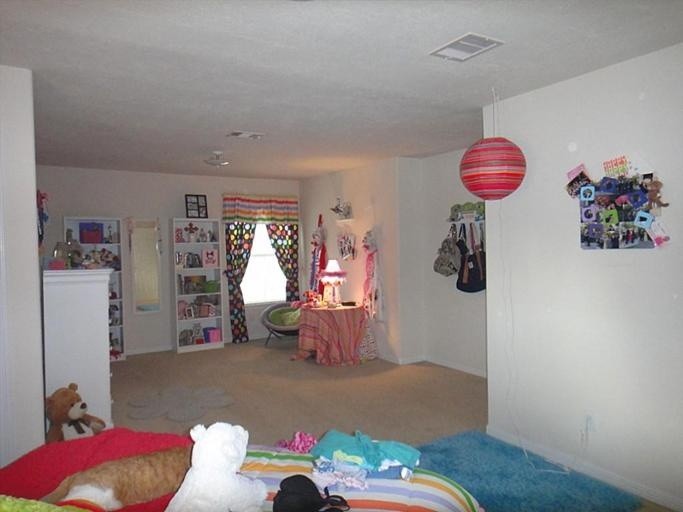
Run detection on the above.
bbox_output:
[291,301,380,367]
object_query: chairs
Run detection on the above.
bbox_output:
[260,302,302,348]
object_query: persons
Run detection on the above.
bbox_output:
[586,209,593,218]
[605,213,613,223]
[581,224,645,246]
[584,191,591,198]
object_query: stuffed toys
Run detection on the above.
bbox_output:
[162,421,267,512]
[291,288,322,310]
[39,444,192,509]
[45,383,106,443]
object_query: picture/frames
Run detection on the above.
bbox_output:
[185,193,208,218]
[129,214,163,316]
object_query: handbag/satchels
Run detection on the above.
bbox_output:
[432,222,485,293]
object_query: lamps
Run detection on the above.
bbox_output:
[203,151,233,168]
[457,83,526,201]
[316,258,346,307]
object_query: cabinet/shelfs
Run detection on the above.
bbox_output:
[170,217,223,355]
[41,266,113,442]
[62,216,126,362]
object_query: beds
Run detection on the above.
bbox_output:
[0,422,483,512]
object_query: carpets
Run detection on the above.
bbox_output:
[397,429,641,511]
